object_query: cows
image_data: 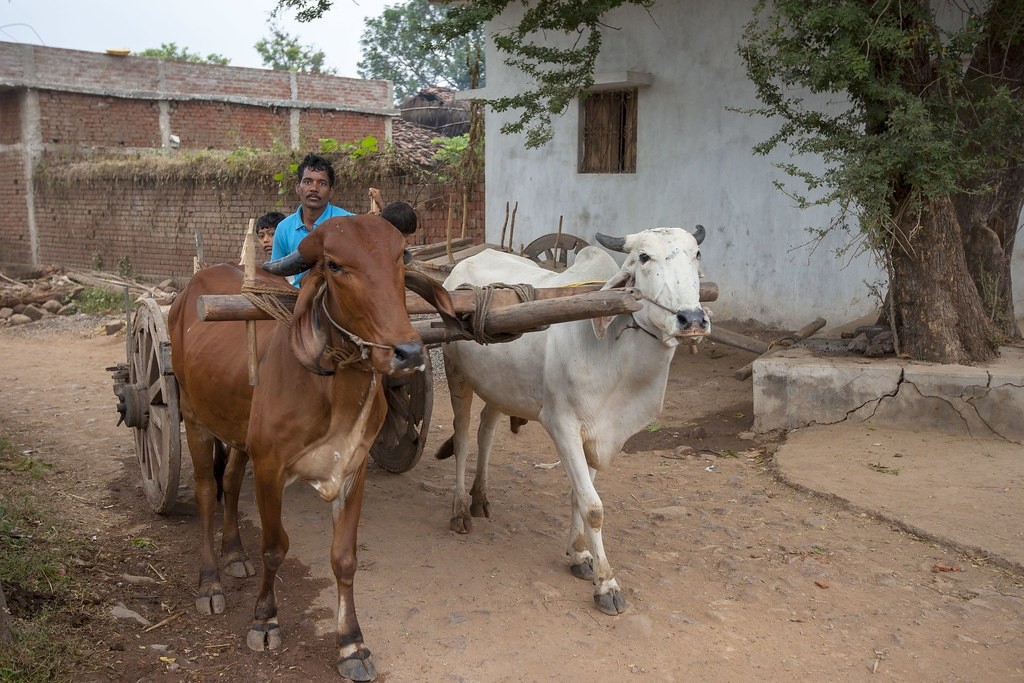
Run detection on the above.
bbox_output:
[167,215,426,683]
[439,223,711,616]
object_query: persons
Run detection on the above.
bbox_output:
[195,211,287,267]
[369,187,417,237]
[269,152,358,291]
